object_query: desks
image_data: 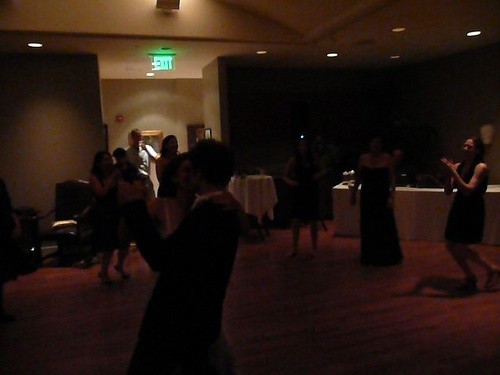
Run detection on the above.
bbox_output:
[229,175,276,243]
[332,181,500,245]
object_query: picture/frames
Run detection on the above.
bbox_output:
[128,130,163,159]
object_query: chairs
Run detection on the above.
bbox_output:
[27,180,93,262]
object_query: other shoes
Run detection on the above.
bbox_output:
[461,274,476,294]
[0,311,16,322]
[286,251,297,258]
[484,265,500,288]
[310,249,318,257]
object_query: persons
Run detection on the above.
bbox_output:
[88,129,242,289]
[282,127,327,258]
[116,138,246,375]
[440,135,500,295]
[351,134,404,272]
[391,144,423,188]
[0,176,22,324]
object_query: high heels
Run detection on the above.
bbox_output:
[115,266,130,280]
[98,272,111,287]
